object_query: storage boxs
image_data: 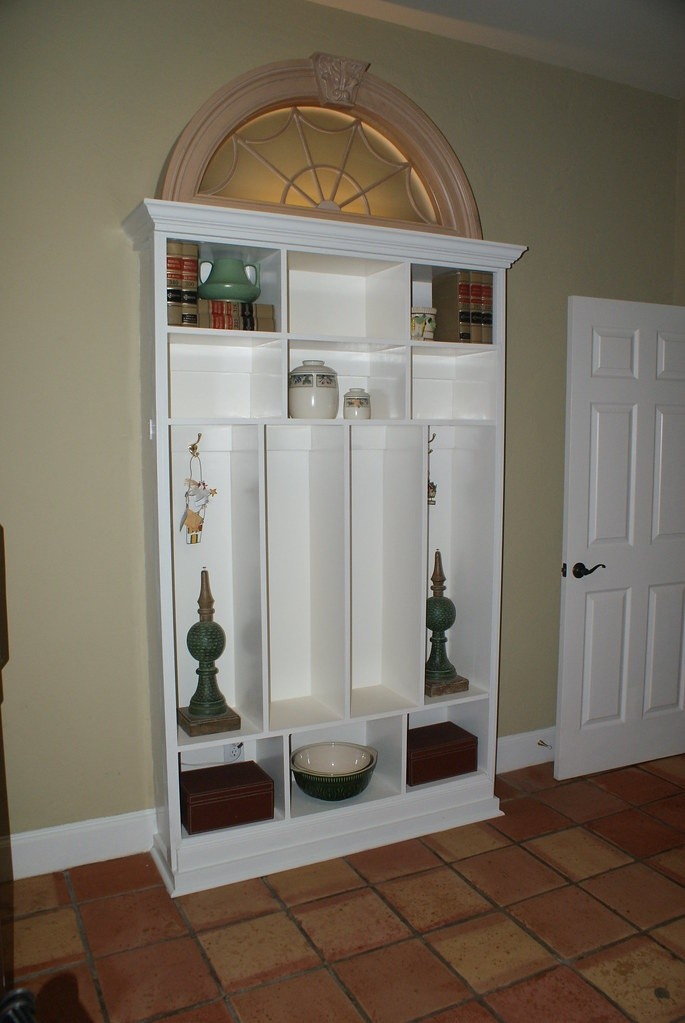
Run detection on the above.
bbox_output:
[407,720,479,787]
[180,760,274,835]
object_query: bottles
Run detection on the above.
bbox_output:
[288,360,339,419]
[343,387,371,420]
[196,257,262,303]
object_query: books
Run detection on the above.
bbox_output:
[197,300,275,331]
[166,240,198,326]
[432,269,493,345]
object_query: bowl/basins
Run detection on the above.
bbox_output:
[291,742,378,803]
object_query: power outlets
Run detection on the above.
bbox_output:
[223,742,245,764]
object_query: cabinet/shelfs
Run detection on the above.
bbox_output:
[120,197,528,900]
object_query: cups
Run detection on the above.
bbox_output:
[411,307,437,341]
